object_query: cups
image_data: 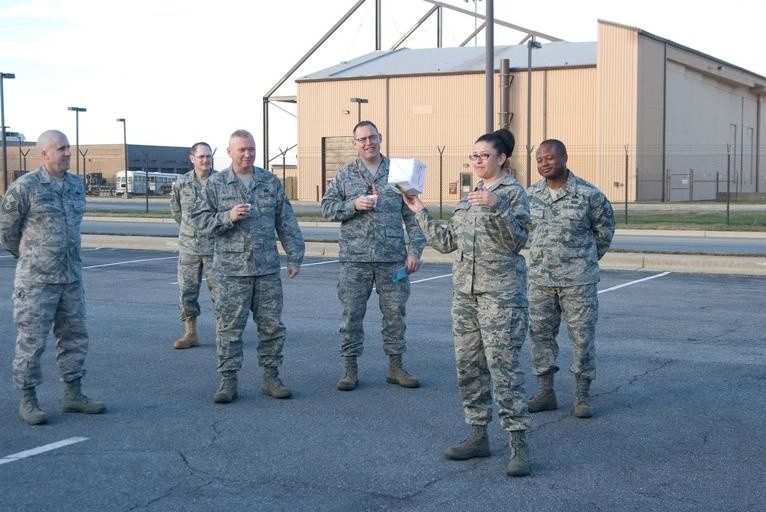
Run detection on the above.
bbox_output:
[367,194,378,208]
[244,203,250,216]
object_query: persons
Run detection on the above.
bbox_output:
[402,129,530,476]
[321,121,431,391]
[0,130,106,424]
[192,130,305,403]
[525,138,616,418]
[170,142,220,349]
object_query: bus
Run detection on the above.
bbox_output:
[115,170,184,196]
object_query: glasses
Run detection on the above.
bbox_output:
[469,153,489,161]
[193,154,211,159]
[355,135,379,143]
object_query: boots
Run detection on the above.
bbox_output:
[173,320,199,349]
[386,355,419,388]
[214,371,238,403]
[506,431,529,476]
[62,377,105,414]
[444,425,490,460]
[19,388,48,424]
[336,356,359,391]
[574,378,593,418]
[526,373,557,413]
[261,366,291,398]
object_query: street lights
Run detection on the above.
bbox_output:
[0,70,15,194]
[116,117,131,199]
[349,96,368,127]
[67,106,87,177]
[524,39,542,188]
